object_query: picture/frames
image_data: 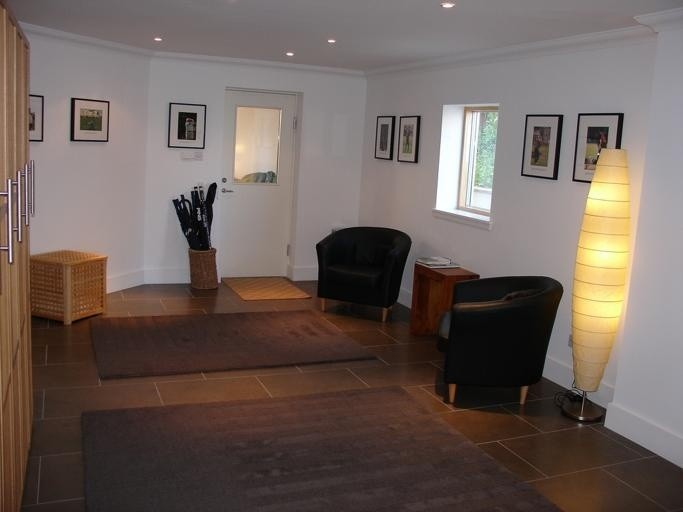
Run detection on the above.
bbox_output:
[397,116,420,162]
[71,98,109,142]
[375,116,395,160]
[521,115,563,180]
[168,103,206,149]
[573,113,624,183]
[29,95,44,142]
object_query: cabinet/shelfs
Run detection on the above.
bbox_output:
[409,260,479,336]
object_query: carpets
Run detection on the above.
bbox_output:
[90,310,377,379]
[221,277,312,301]
[82,387,564,512]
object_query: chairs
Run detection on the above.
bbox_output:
[438,276,563,404]
[316,227,411,322]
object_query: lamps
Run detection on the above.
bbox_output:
[562,149,632,422]
